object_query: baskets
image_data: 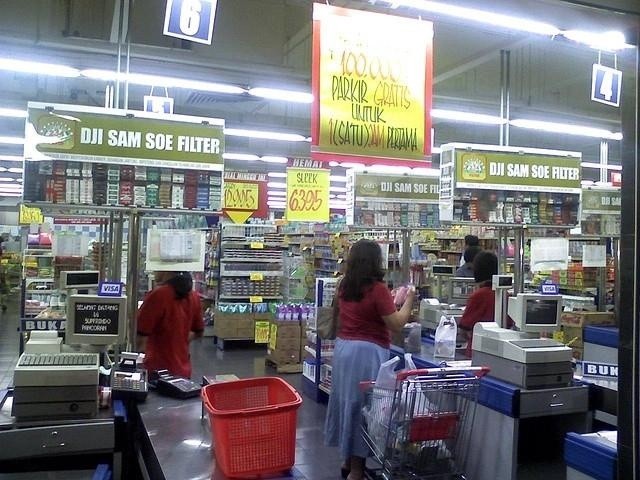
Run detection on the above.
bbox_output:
[201,376,302,479]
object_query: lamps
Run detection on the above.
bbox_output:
[562,29,627,52]
[327,159,441,176]
[0,61,310,170]
[430,109,623,170]
[387,2,559,37]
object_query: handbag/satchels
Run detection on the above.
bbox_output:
[315,276,346,341]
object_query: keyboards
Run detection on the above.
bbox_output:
[509,340,566,348]
[445,309,466,315]
[19,353,97,366]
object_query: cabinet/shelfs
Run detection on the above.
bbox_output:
[1,155,622,373]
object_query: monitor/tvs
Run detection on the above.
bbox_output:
[448,277,476,303]
[508,293,562,333]
[67,295,127,351]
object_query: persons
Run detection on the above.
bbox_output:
[320,238,417,480]
[127,270,205,381]
[455,235,479,271]
[452,246,484,278]
[458,251,516,362]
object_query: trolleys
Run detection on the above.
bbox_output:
[356,361,492,479]
